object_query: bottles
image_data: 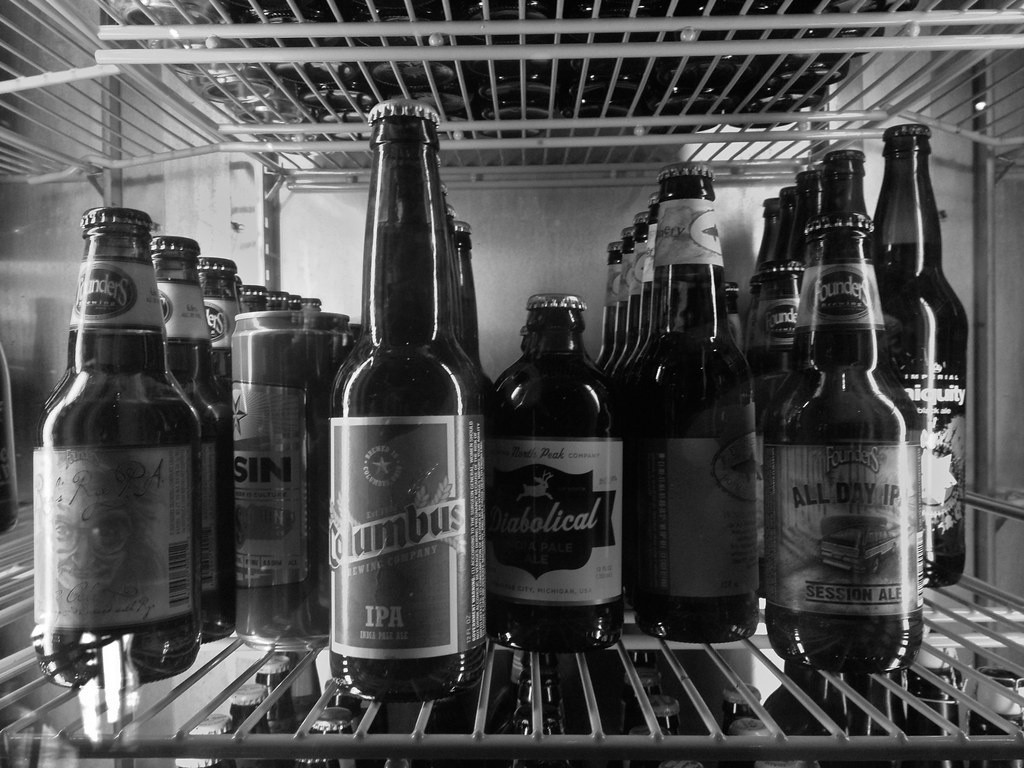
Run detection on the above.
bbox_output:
[175,650,1024,767]
[597,163,762,642]
[749,124,972,671]
[121,0,919,146]
[489,292,622,649]
[33,205,323,684]
[325,100,495,702]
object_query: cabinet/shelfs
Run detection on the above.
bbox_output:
[0,0,1024,768]
